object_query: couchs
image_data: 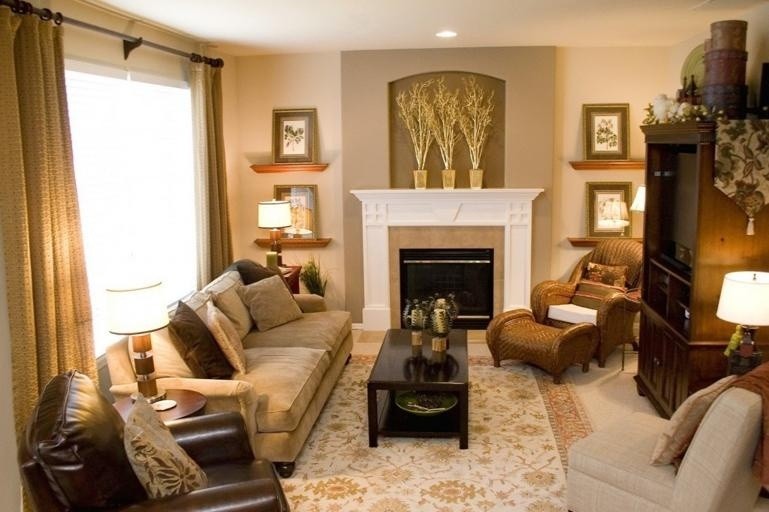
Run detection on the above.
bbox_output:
[105,258,352,480]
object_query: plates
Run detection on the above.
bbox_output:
[151,399,177,411]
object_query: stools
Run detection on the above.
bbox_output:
[486,309,600,384]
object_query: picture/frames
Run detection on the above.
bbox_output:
[582,103,633,238]
[272,107,319,240]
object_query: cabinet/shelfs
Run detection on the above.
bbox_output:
[570,160,647,248]
[632,119,769,420]
[250,162,331,249]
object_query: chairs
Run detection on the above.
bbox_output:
[530,240,647,368]
[18,370,291,512]
[566,362,768,512]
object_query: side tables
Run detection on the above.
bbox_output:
[280,264,304,294]
[115,388,208,420]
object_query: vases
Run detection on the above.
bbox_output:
[414,169,483,189]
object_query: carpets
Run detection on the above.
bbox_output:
[274,355,594,511]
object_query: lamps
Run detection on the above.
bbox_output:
[105,280,171,405]
[629,187,646,212]
[715,271,769,376]
[257,200,292,268]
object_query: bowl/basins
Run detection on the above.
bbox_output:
[395,391,458,417]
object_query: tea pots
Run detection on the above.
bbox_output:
[653,92,674,120]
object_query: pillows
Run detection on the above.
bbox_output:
[124,393,208,498]
[648,373,739,467]
[127,270,304,379]
[572,238,642,311]
